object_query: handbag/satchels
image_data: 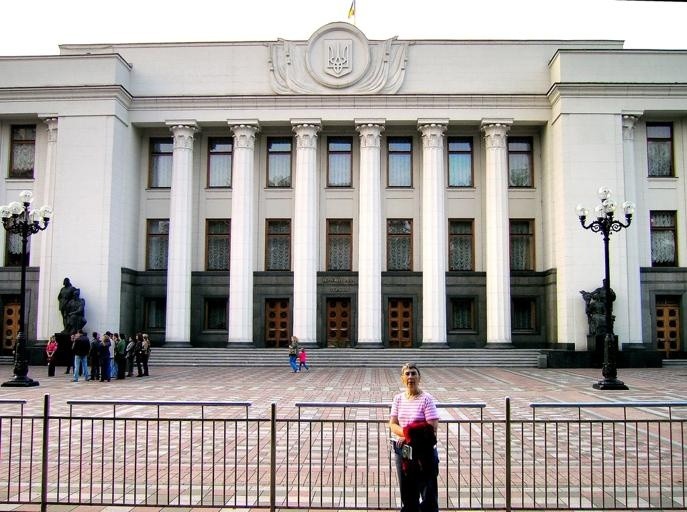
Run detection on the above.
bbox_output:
[289,345,297,357]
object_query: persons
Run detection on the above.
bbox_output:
[65,328,152,382]
[58,277,76,324]
[288,335,299,372]
[583,293,608,332]
[64,289,87,334]
[296,348,310,373]
[45,335,60,377]
[387,361,441,512]
[590,279,616,315]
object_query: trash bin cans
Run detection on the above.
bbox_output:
[537,355,548,368]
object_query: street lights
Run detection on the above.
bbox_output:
[576,188,634,389]
[2,190,53,387]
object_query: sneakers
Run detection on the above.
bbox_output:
[290,369,309,373]
[64,370,149,383]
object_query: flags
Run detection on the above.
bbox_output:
[348,0,355,18]
